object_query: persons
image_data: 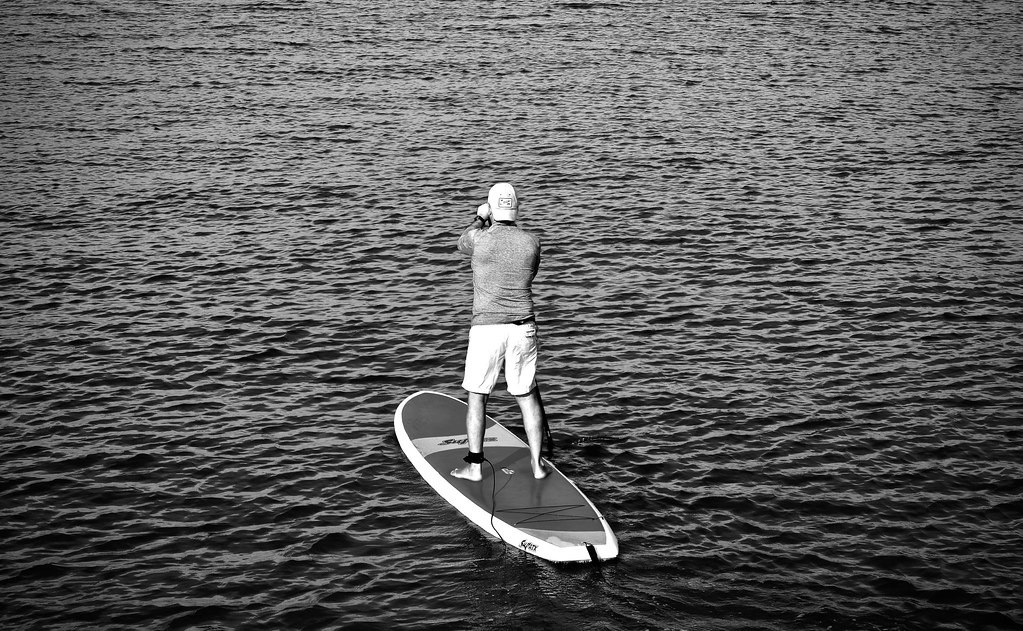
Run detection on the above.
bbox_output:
[450,182,552,482]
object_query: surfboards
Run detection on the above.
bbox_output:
[394,389,620,564]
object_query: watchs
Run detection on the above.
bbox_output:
[474,216,485,227]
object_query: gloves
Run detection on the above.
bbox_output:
[477,203,491,220]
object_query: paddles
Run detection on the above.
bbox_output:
[486,214,554,453]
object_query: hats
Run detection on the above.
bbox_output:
[488,183,519,221]
